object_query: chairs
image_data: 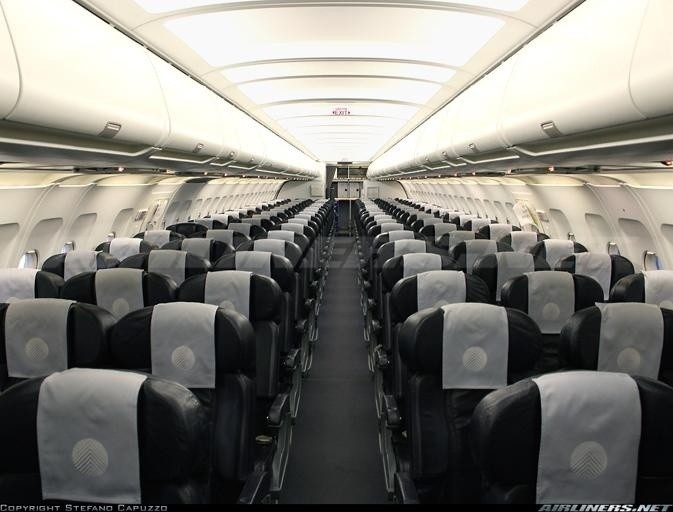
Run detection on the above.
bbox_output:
[443,369,673,511]
[2,369,211,507]
[392,302,550,498]
[2,297,114,380]
[110,301,265,488]
[557,300,673,389]
